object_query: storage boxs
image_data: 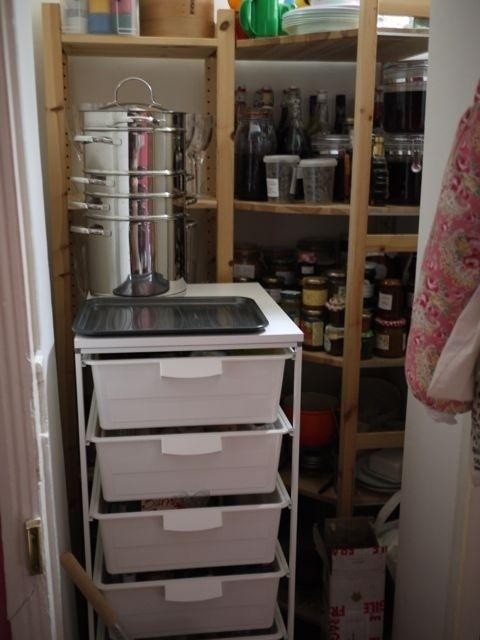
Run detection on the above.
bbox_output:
[85,353,292,640]
[310,512,389,639]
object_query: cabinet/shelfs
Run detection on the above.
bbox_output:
[73,279,306,639]
[237,0,429,577]
[40,2,236,488]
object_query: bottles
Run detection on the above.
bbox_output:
[58,0,142,37]
[235,59,429,204]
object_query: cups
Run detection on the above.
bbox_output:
[239,0,280,37]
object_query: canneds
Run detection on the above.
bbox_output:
[237,235,410,360]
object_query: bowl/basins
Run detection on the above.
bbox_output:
[283,388,340,448]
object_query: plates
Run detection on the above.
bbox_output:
[355,451,404,495]
[282,2,365,34]
